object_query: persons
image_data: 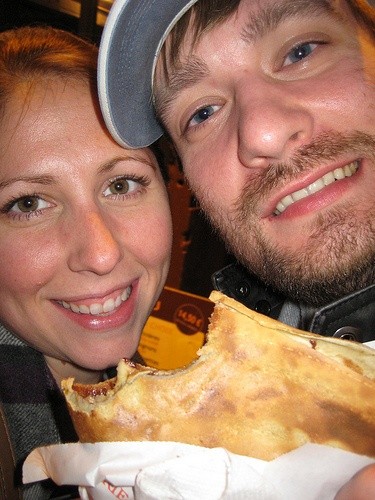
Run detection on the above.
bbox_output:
[0,25,177,499]
[96,0,375,500]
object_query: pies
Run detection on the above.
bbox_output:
[61,289,375,461]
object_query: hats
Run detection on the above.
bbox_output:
[98,0,199,151]
